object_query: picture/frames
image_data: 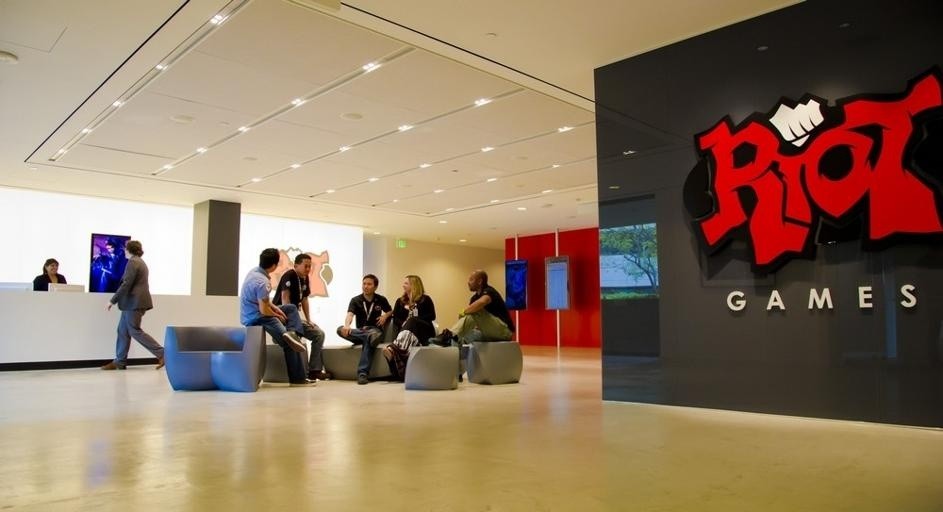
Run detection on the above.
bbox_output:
[700,237,777,289]
[90,233,131,292]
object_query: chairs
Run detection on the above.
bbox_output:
[163,324,267,393]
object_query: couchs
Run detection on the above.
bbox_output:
[320,318,438,379]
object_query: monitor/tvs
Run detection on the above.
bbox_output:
[48,283,85,292]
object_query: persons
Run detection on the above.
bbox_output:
[98,239,167,370]
[269,253,332,381]
[30,258,66,291]
[381,273,436,384]
[238,247,318,388]
[96,242,118,294]
[335,273,393,384]
[428,269,517,383]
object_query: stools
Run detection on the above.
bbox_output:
[263,343,308,382]
[404,346,459,391]
[466,340,523,385]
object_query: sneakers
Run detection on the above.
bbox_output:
[102,361,126,371]
[383,347,394,361]
[307,369,333,379]
[370,332,383,345]
[358,373,368,384]
[283,330,306,353]
[428,328,451,347]
[156,354,164,370]
[289,378,316,388]
